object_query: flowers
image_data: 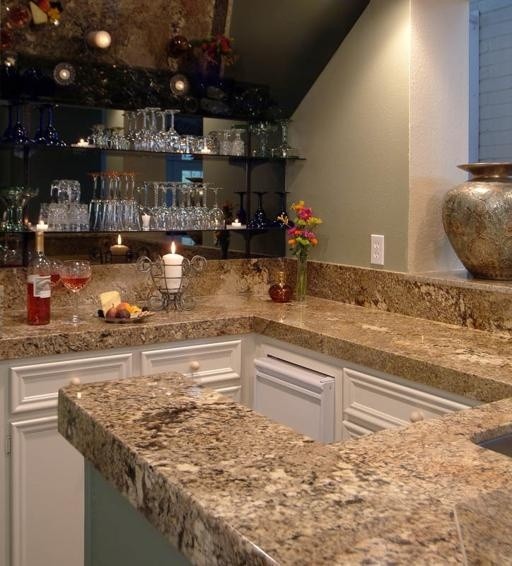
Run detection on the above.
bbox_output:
[189,29,242,68]
[272,197,325,296]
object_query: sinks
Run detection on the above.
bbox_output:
[467,422,512,460]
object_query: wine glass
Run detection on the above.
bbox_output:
[10,100,64,146]
[61,259,92,325]
[88,169,227,231]
[234,191,247,225]
[251,191,271,228]
[88,106,297,157]
[1,188,39,233]
[273,191,291,227]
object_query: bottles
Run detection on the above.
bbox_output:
[268,271,293,303]
[27,231,52,326]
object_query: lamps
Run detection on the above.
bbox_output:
[85,28,111,49]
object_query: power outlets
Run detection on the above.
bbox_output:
[370,235,386,266]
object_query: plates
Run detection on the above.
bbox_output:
[93,311,156,323]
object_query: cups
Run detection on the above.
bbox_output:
[40,179,92,231]
[47,259,60,289]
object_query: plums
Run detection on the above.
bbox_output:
[118,310,130,318]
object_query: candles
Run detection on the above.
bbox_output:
[34,220,50,231]
[76,136,90,146]
[107,232,131,264]
[139,211,153,227]
[198,146,212,153]
[157,239,184,293]
[229,217,242,227]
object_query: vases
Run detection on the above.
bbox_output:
[293,253,309,303]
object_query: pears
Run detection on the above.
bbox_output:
[106,307,118,318]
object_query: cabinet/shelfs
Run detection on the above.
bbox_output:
[0,341,136,565]
[237,340,343,448]
[0,121,305,248]
[133,330,251,412]
[334,357,479,452]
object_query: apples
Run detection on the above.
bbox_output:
[117,302,129,310]
